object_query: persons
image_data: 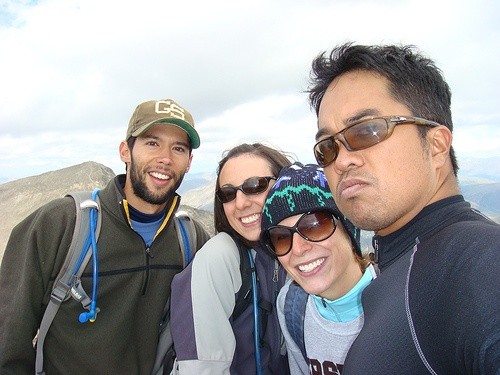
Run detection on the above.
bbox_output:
[259,162,379,375]
[158,142,296,375]
[307,41,500,375]
[0,99,212,375]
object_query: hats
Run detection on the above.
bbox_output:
[261,161,363,258]
[125,99,200,149]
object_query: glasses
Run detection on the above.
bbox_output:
[260,209,339,256]
[216,176,275,203]
[313,116,439,167]
